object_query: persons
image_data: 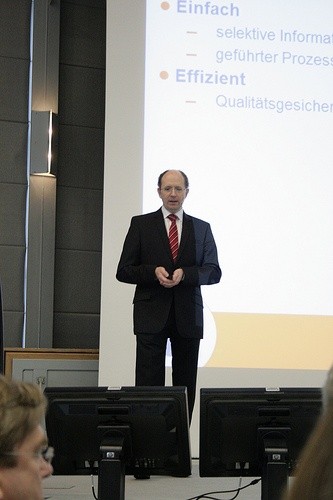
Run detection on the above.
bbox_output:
[0,373,54,500]
[116,170,222,428]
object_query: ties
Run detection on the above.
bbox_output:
[166,214,179,263]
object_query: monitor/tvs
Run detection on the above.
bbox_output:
[44,386,192,500]
[199,388,323,500]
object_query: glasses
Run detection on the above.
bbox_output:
[4,447,53,464]
[161,186,186,192]
